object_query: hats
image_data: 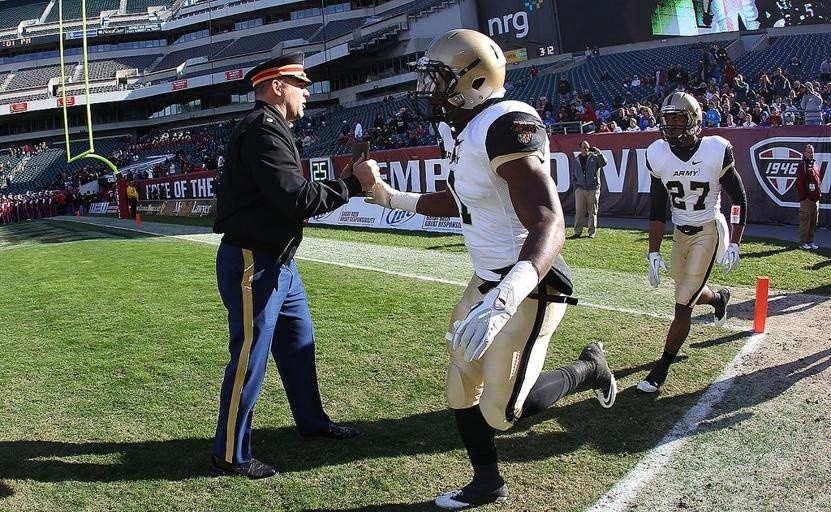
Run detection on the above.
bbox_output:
[243,51,313,89]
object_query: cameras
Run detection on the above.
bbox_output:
[589,147,595,151]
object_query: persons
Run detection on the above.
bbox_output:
[634,91,747,394]
[210,50,381,479]
[359,28,617,509]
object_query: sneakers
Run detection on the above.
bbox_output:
[799,243,811,250]
[810,243,818,250]
[323,422,361,440]
[589,233,596,237]
[571,234,582,239]
[638,364,669,392]
[579,342,619,409]
[211,455,276,481]
[715,288,729,328]
[435,478,507,509]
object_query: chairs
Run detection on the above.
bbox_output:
[504,32,831,125]
[2,1,439,194]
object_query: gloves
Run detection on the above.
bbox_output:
[720,243,740,272]
[646,251,668,287]
[364,179,423,213]
[452,260,539,363]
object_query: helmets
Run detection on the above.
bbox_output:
[407,27,507,117]
[660,91,703,145]
